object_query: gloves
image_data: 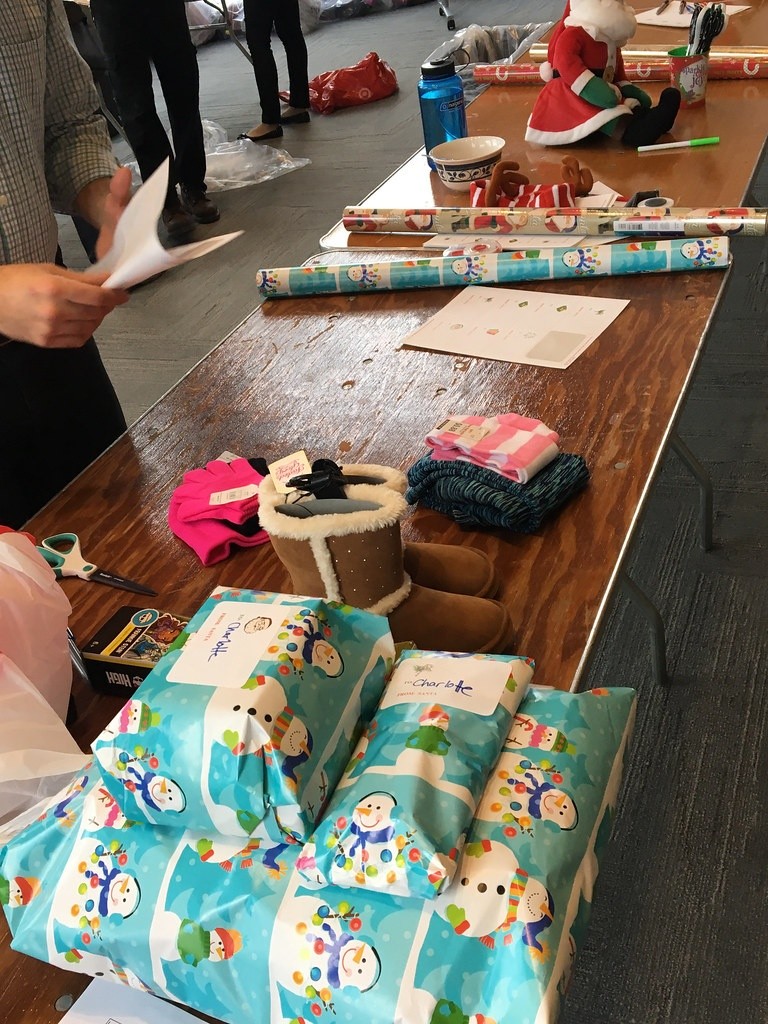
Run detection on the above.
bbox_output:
[174,457,267,524]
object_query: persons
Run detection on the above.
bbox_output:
[0,0,133,533]
[89,0,221,238]
[525,0,680,150]
[236,0,311,142]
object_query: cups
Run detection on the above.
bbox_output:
[667,45,711,110]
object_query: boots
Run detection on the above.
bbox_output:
[159,185,196,237]
[256,462,499,600]
[257,482,509,656]
[181,183,221,223]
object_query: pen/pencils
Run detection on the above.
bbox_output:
[656,0,703,16]
[637,136,720,153]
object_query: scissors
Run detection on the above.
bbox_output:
[686,2,729,56]
[34,531,159,598]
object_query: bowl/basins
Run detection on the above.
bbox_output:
[428,135,506,192]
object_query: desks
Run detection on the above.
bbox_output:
[320,0,768,559]
[0,249,732,1024]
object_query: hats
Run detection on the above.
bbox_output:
[168,488,272,565]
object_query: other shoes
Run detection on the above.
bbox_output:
[280,111,310,125]
[237,126,283,142]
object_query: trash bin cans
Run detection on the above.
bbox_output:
[420,20,556,109]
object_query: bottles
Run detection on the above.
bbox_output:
[417,48,471,172]
